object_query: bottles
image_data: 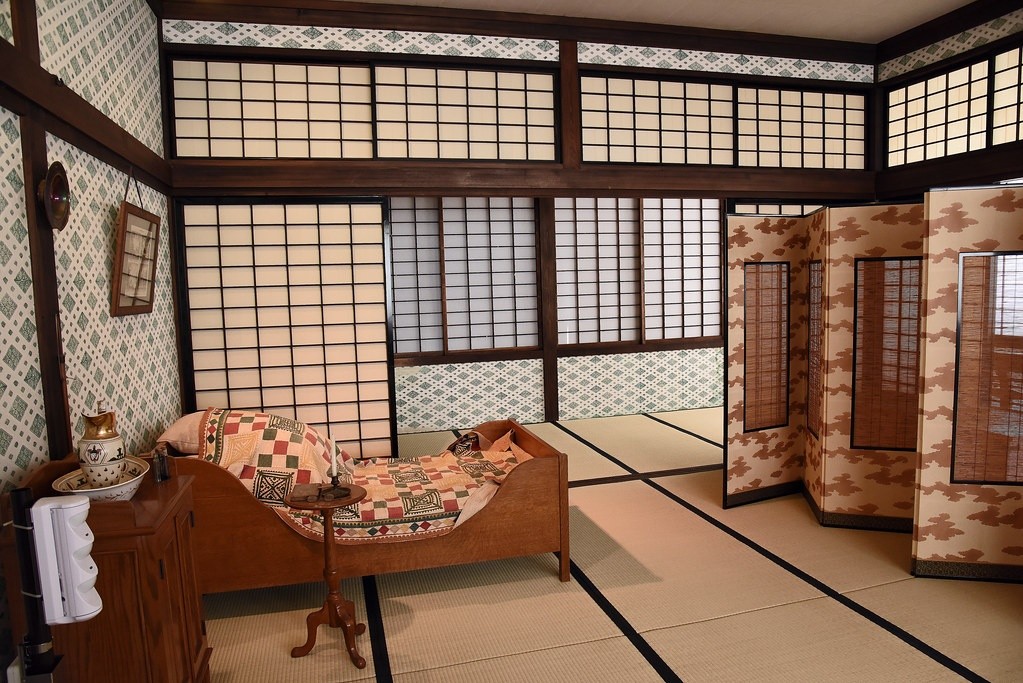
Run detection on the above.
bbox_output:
[153,446,170,483]
[77,411,124,488]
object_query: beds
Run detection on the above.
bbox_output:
[137,407,573,597]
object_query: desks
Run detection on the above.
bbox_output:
[283,483,368,670]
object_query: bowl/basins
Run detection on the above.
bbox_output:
[52,457,150,501]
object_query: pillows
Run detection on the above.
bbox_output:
[154,411,322,456]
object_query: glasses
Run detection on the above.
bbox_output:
[291,487,334,503]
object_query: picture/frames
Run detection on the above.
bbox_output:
[110,200,161,318]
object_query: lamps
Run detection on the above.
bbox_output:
[38,161,71,234]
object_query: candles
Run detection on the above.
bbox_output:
[330,434,338,477]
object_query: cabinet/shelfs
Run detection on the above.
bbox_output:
[0,452,213,683]
